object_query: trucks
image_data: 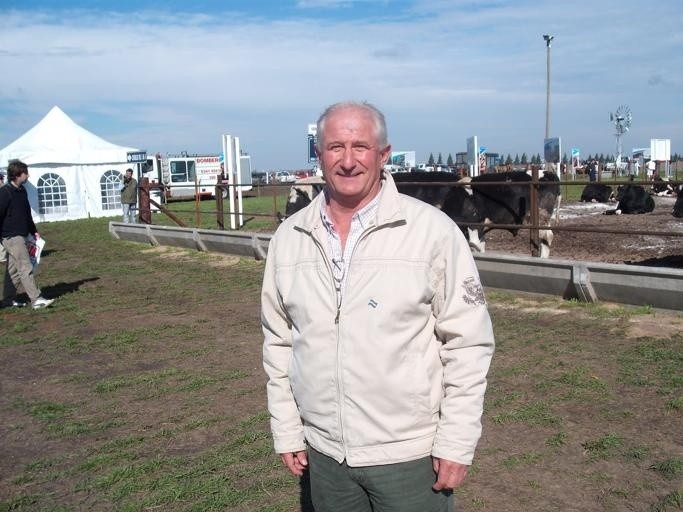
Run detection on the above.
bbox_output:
[138,151,254,203]
[605,156,632,172]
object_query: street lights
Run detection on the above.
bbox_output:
[540,34,557,170]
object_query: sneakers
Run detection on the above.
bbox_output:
[2,297,27,307]
[31,296,55,310]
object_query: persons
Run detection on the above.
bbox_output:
[259,98,499,512]
[588,159,596,182]
[0,160,55,311]
[0,173,8,264]
[118,167,138,224]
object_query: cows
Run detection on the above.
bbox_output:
[581,174,683,218]
[391,172,462,208]
[440,169,562,259]
[276,176,328,225]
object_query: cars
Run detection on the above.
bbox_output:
[583,161,599,174]
[261,167,324,186]
[383,161,458,177]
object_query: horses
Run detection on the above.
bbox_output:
[493,163,510,173]
